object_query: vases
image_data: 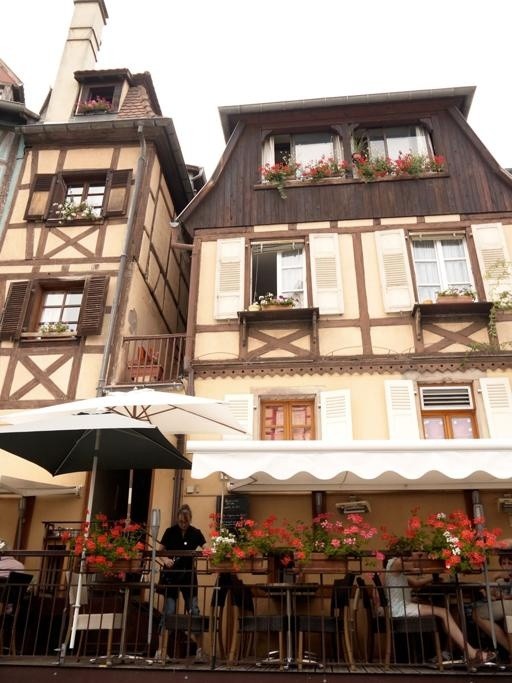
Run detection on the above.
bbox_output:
[261,304,292,311]
[128,366,164,382]
[85,551,143,574]
[436,295,473,304]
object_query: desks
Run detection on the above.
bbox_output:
[423,580,506,672]
[0,567,445,672]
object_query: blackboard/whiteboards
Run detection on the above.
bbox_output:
[217,495,250,543]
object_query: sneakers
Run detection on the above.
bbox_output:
[433,652,452,666]
[152,650,170,664]
[195,648,207,661]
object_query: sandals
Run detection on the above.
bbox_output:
[468,648,500,668]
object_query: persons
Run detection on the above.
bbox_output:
[155,506,212,663]
[429,554,512,668]
[0,540,25,654]
[383,539,500,666]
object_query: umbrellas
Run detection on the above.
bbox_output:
[37,385,249,532]
[0,410,191,656]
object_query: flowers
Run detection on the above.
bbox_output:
[40,320,70,334]
[60,507,147,584]
[258,292,299,307]
[51,200,100,224]
[129,349,161,365]
[76,94,114,114]
[435,283,479,299]
[201,504,506,575]
[256,146,447,200]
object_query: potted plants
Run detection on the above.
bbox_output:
[350,127,370,159]
